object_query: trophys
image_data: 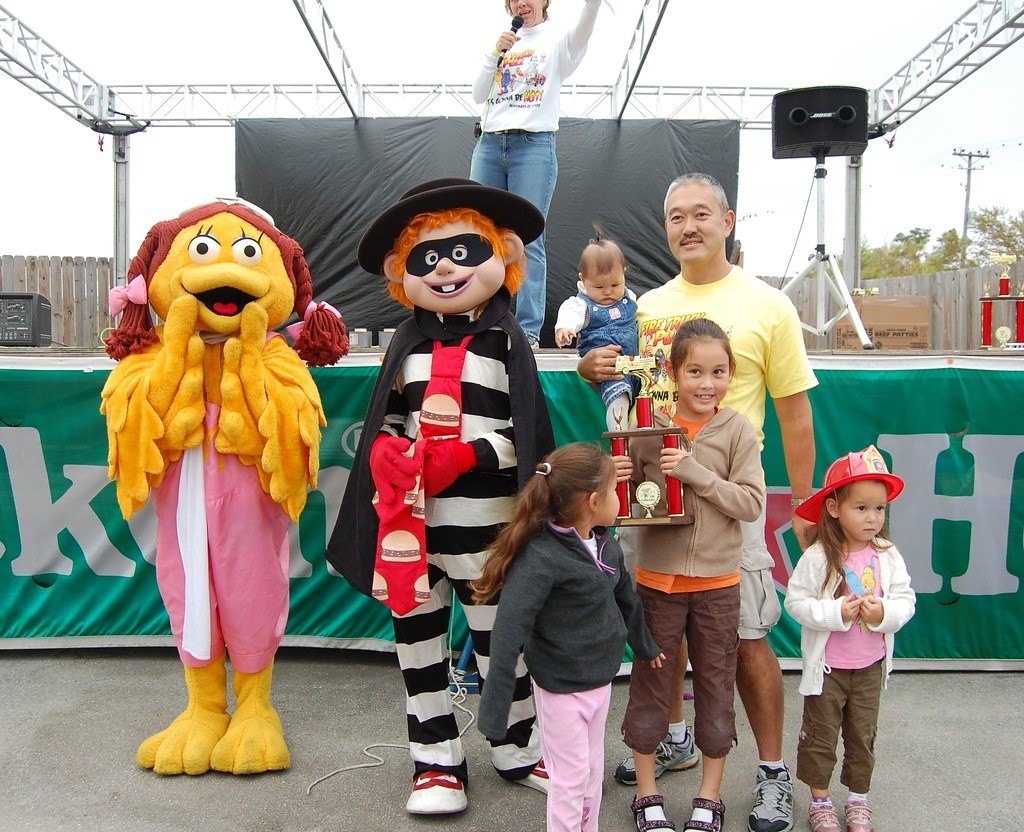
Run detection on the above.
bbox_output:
[602,388,694,525]
[980,272,1024,351]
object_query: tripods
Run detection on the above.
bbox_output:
[779,156,874,350]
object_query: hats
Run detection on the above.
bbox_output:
[796,445,905,524]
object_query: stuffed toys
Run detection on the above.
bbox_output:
[101,198,350,778]
[324,177,557,816]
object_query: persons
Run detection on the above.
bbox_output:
[610,319,766,832]
[468,443,667,832]
[579,173,815,832]
[784,445,917,832]
[470,0,601,354]
[554,222,642,432]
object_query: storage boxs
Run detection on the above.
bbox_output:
[836,294,930,349]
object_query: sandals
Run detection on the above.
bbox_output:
[631,791,677,832]
[683,797,725,832]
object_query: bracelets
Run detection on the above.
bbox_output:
[791,494,813,506]
[576,358,593,383]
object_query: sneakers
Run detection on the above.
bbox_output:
[809,802,875,832]
[614,727,700,786]
[747,765,794,832]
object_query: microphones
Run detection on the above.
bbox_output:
[497,15,524,67]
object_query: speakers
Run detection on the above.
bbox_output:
[771,86,868,159]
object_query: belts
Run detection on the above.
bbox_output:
[494,129,525,135]
